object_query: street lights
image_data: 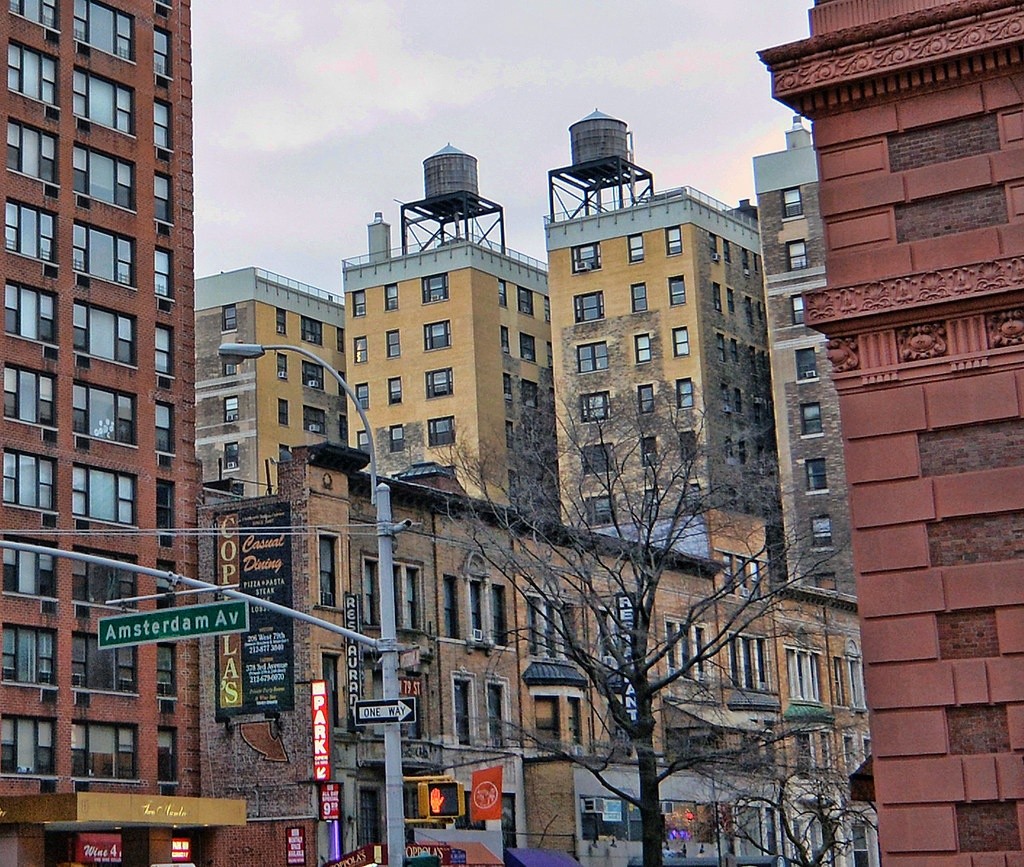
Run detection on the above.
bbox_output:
[217,339,415,866]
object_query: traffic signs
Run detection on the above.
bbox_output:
[354,697,418,726]
[97,598,252,652]
[400,647,422,669]
[397,676,423,696]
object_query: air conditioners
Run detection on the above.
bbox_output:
[658,801,675,815]
[580,798,606,814]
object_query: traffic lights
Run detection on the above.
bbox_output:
[417,780,468,820]
